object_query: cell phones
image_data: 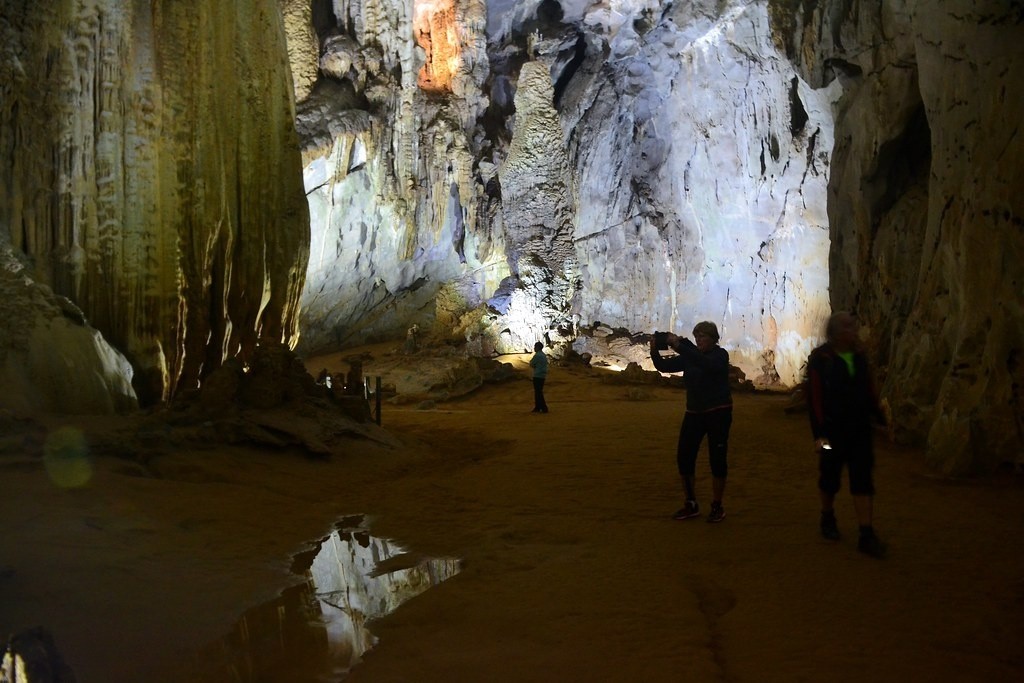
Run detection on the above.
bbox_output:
[823,444,833,450]
[655,331,669,350]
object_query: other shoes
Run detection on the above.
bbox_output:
[542,407,548,413]
[857,530,890,560]
[820,509,840,540]
[672,499,700,520]
[531,407,541,412]
[707,503,727,523]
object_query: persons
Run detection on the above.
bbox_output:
[807,311,891,556]
[530,341,547,414]
[650,321,733,522]
